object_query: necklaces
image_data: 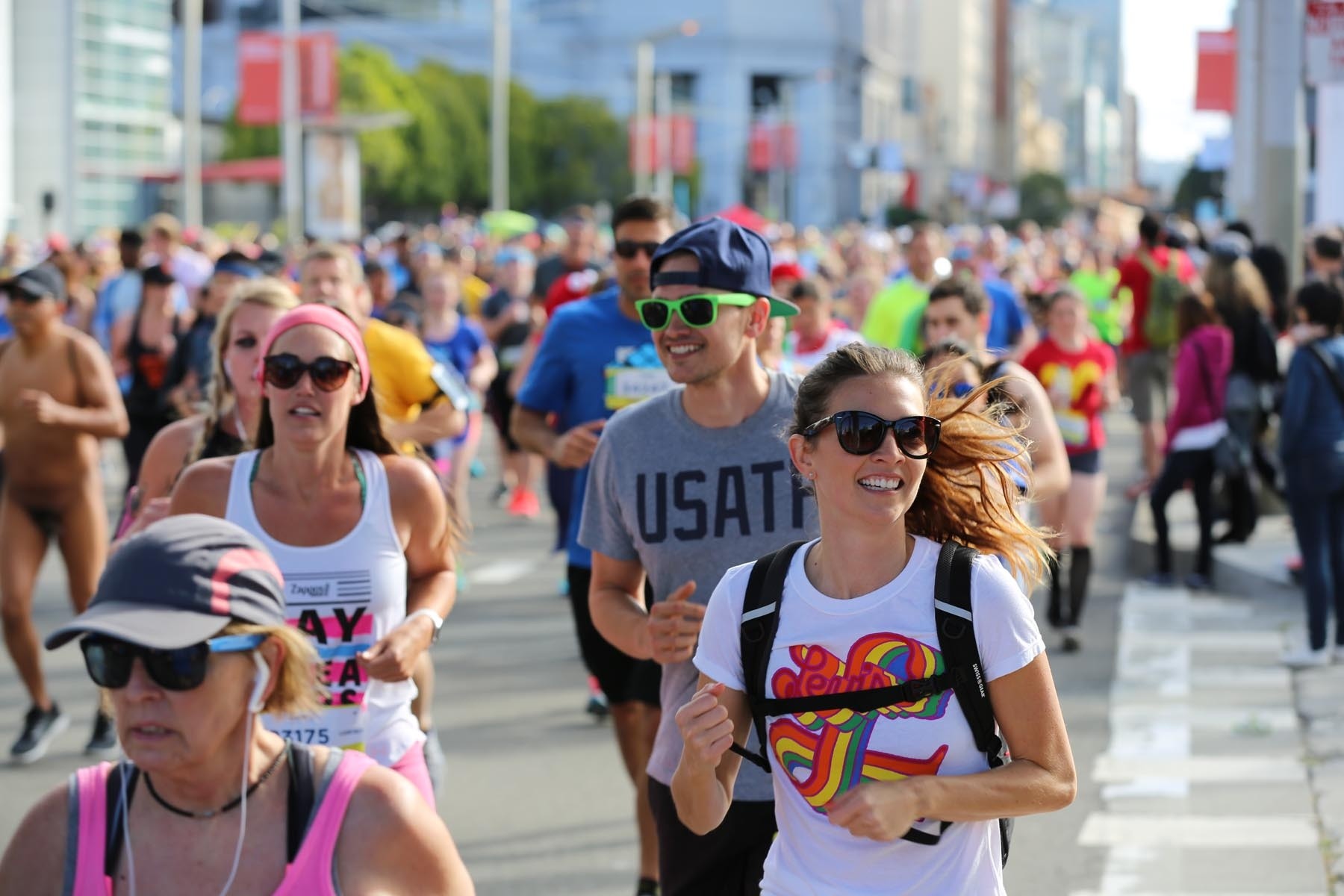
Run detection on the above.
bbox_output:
[233,401,253,452]
[141,737,292,822]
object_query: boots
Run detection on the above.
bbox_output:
[1070,545,1091,626]
[1044,551,1063,631]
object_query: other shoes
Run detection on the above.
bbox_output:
[635,876,660,896]
[587,693,608,718]
[1280,637,1331,665]
[1185,571,1214,593]
[85,709,120,761]
[1142,563,1173,587]
[10,701,68,765]
[507,486,539,518]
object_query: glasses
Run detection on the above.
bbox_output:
[79,630,266,692]
[614,239,660,260]
[263,353,359,393]
[6,287,44,306]
[800,410,942,459]
[927,380,974,398]
[634,293,757,331]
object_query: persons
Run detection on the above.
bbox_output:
[509,196,819,896]
[0,218,459,817]
[353,202,1344,668]
[671,343,1074,896]
[0,513,479,896]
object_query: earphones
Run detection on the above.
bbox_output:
[245,650,267,713]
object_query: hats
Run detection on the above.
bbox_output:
[3,266,68,303]
[650,216,801,317]
[43,513,284,651]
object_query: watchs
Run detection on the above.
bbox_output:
[405,608,442,646]
